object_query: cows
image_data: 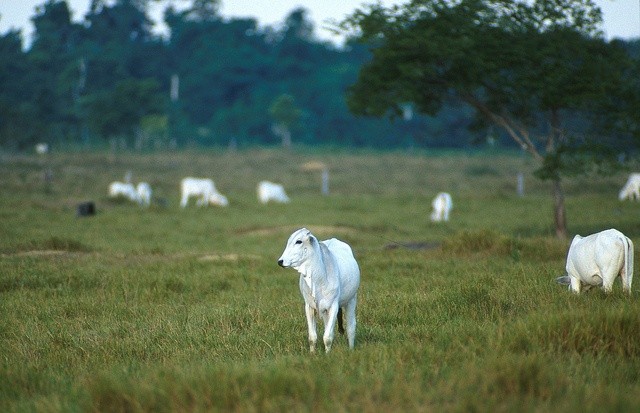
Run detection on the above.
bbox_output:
[179,176,216,209]
[429,192,453,222]
[554,227,635,296]
[276,226,361,355]
[618,172,640,202]
